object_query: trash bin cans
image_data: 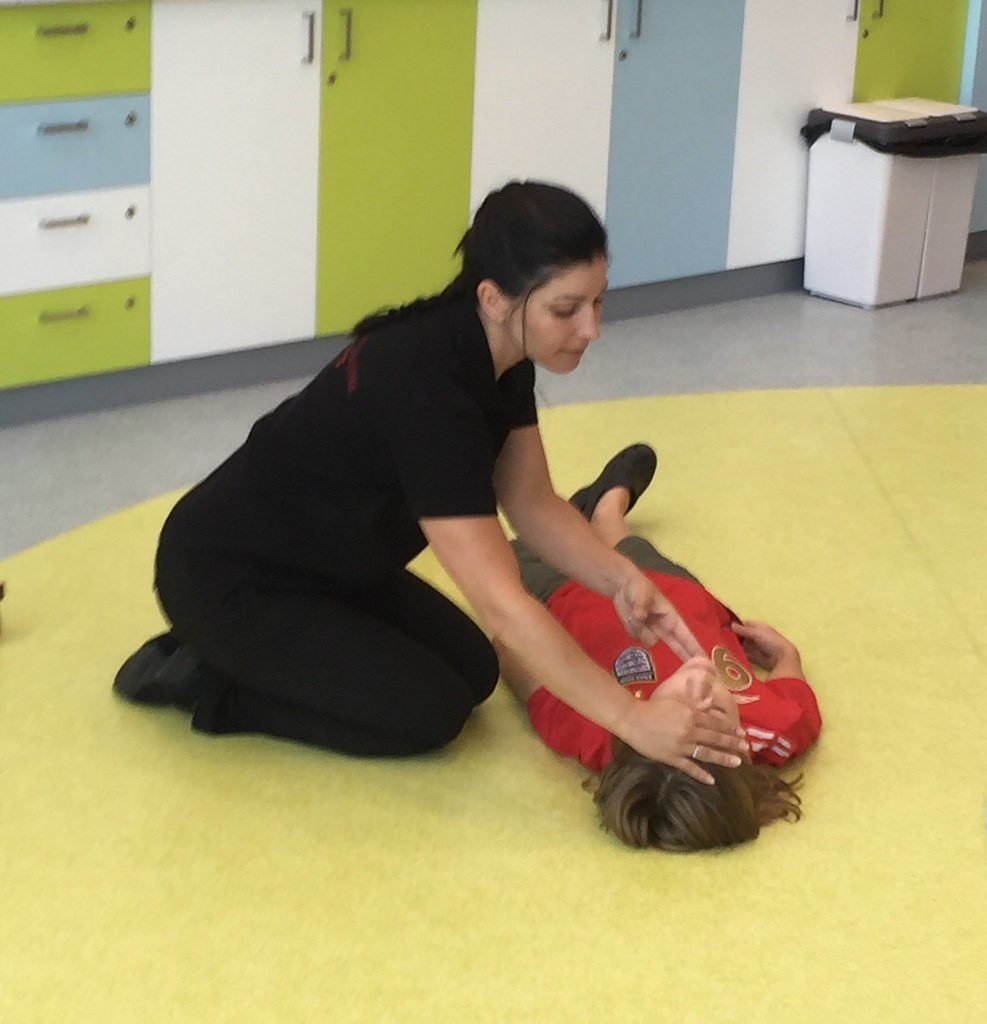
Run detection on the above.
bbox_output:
[800,97,987,312]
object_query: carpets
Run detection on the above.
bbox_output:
[4,383,987,1024]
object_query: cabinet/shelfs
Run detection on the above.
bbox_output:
[0,0,987,392]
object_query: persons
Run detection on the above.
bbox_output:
[489,440,823,854]
[108,176,756,789]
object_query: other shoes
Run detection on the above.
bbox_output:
[567,444,657,527]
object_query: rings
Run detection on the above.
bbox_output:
[693,746,699,758]
